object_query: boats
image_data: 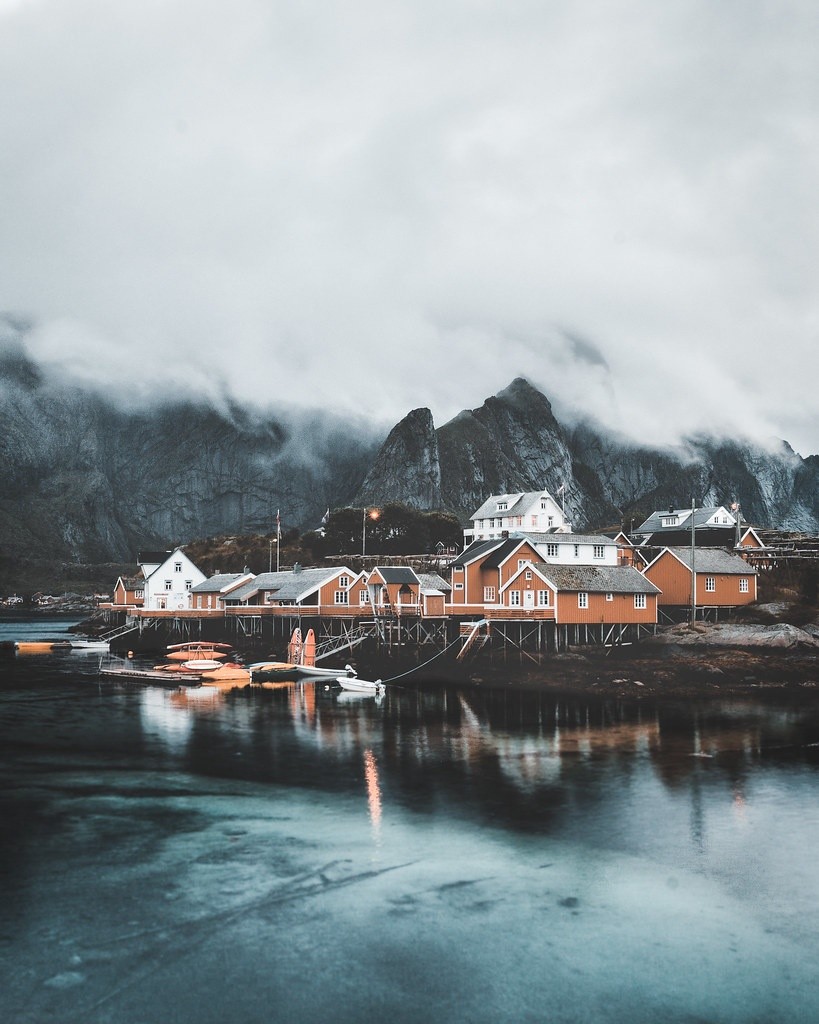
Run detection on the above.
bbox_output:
[14,639,111,652]
[152,627,387,694]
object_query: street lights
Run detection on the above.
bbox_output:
[363,507,379,555]
